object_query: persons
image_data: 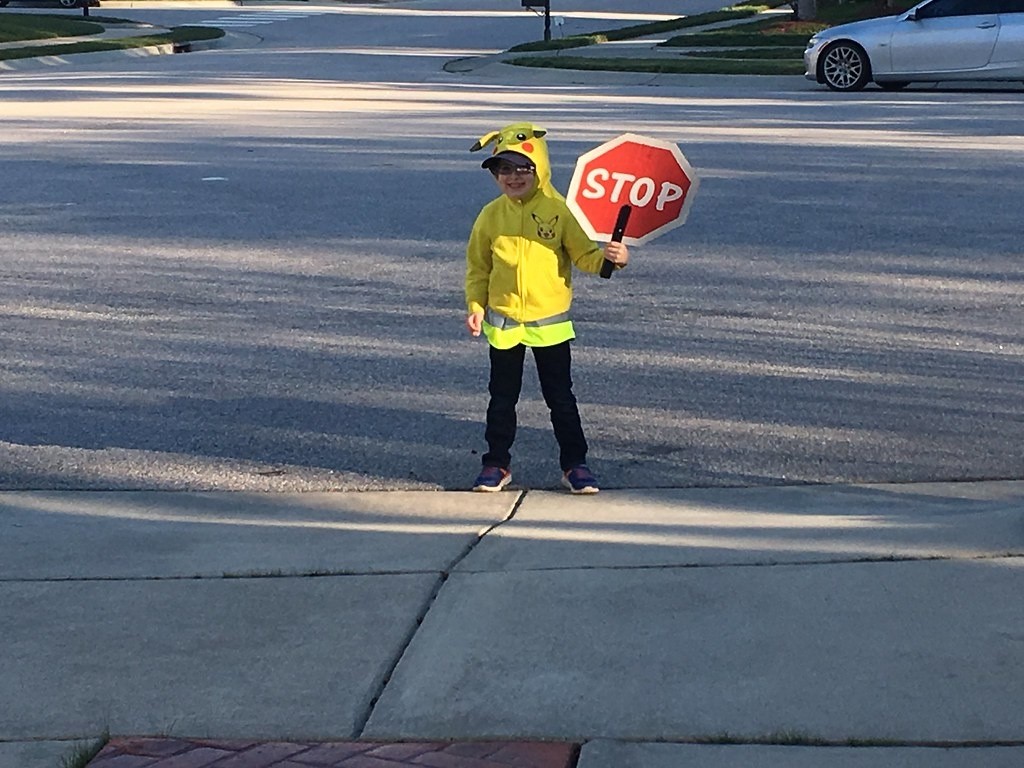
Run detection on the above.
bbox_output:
[465,122,630,494]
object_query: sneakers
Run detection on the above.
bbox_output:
[472,465,512,493]
[561,463,599,493]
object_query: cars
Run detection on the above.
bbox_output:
[802,2,1023,92]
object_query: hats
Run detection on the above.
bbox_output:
[481,152,532,169]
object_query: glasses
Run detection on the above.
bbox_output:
[494,164,535,176]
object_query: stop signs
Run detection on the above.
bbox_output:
[566,132,701,250]
